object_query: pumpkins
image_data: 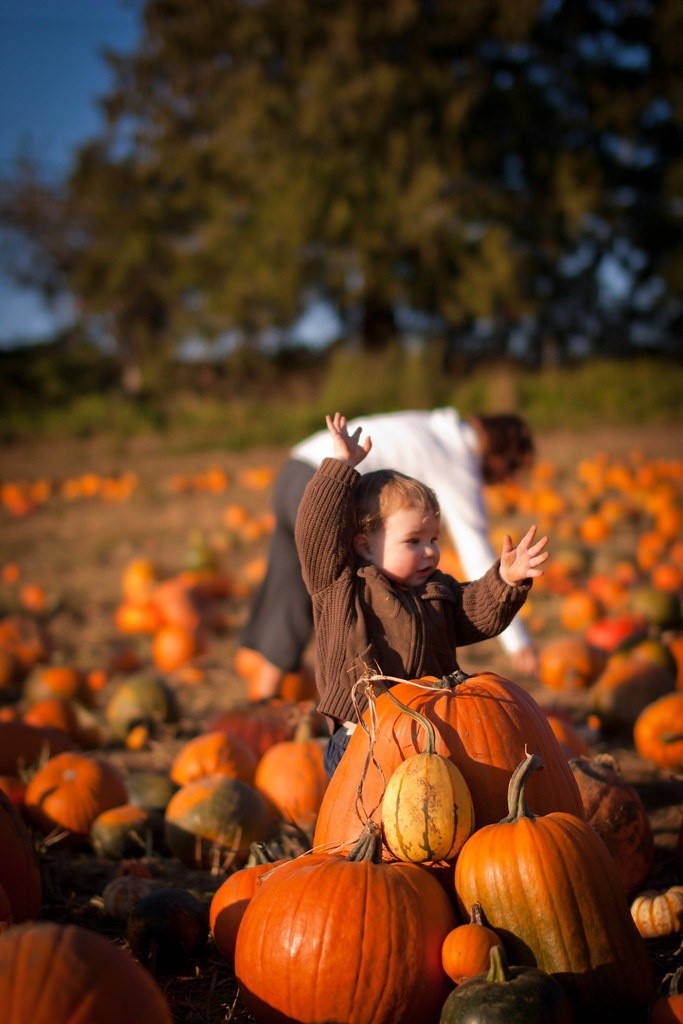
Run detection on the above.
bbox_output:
[2,455,682,1024]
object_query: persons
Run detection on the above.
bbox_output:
[294,411,551,779]
[246,404,540,706]
